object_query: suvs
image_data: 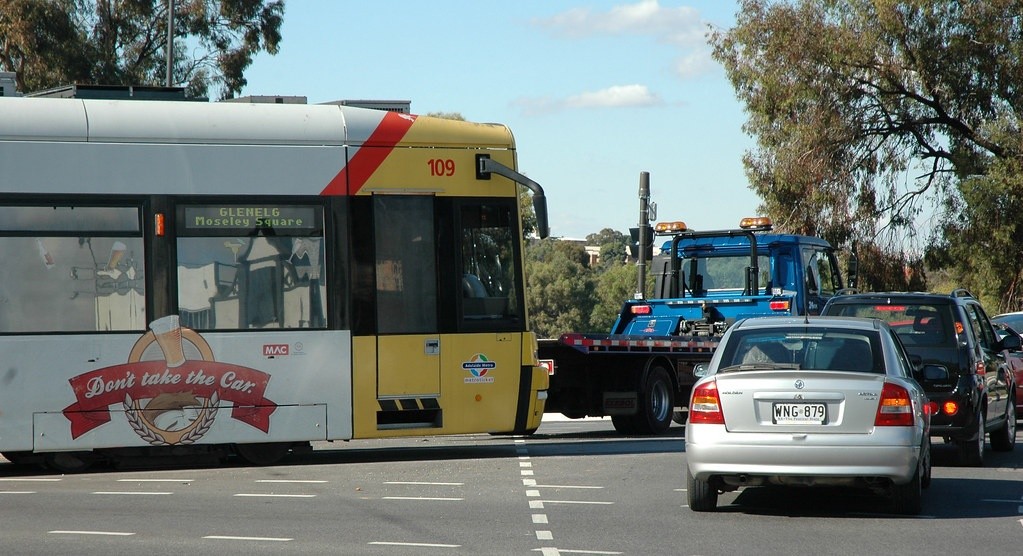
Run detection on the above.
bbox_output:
[802,287,1022,468]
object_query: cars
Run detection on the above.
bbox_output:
[990,310,1023,336]
[991,322,1023,420]
[683,315,949,514]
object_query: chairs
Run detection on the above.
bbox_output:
[745,343,794,366]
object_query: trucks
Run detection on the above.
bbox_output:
[535,217,860,435]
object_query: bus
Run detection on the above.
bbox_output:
[0,68,550,473]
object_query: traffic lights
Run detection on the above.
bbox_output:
[629,227,639,259]
